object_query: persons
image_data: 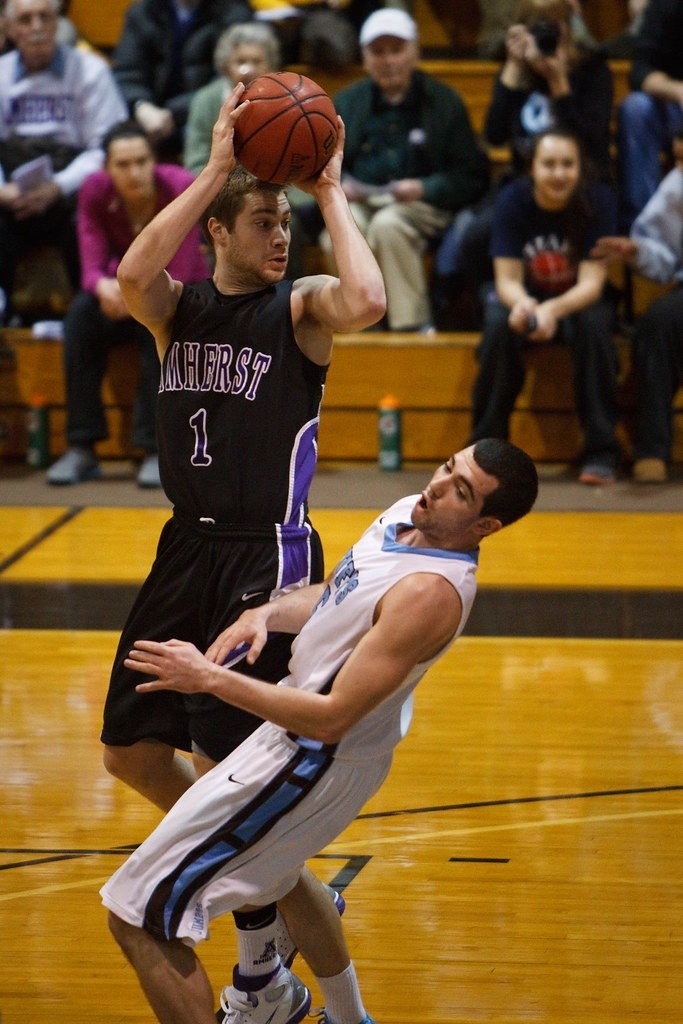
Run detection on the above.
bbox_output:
[44,121,209,487]
[465,126,622,483]
[183,24,315,210]
[100,83,387,1024]
[482,1,614,183]
[0,0,128,322]
[617,0,683,221]
[598,131,683,480]
[113,0,406,165]
[98,439,539,1024]
[319,8,489,330]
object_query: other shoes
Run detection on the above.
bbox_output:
[44,445,99,484]
[136,454,161,487]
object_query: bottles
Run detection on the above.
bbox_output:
[375,395,403,471]
[23,393,54,474]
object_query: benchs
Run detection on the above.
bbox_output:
[1,0,683,465]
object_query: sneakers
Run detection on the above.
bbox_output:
[215,963,311,1024]
[632,457,667,482]
[274,881,345,970]
[578,457,618,484]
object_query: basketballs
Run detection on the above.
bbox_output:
[233,71,339,186]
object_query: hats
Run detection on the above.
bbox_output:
[358,7,418,47]
